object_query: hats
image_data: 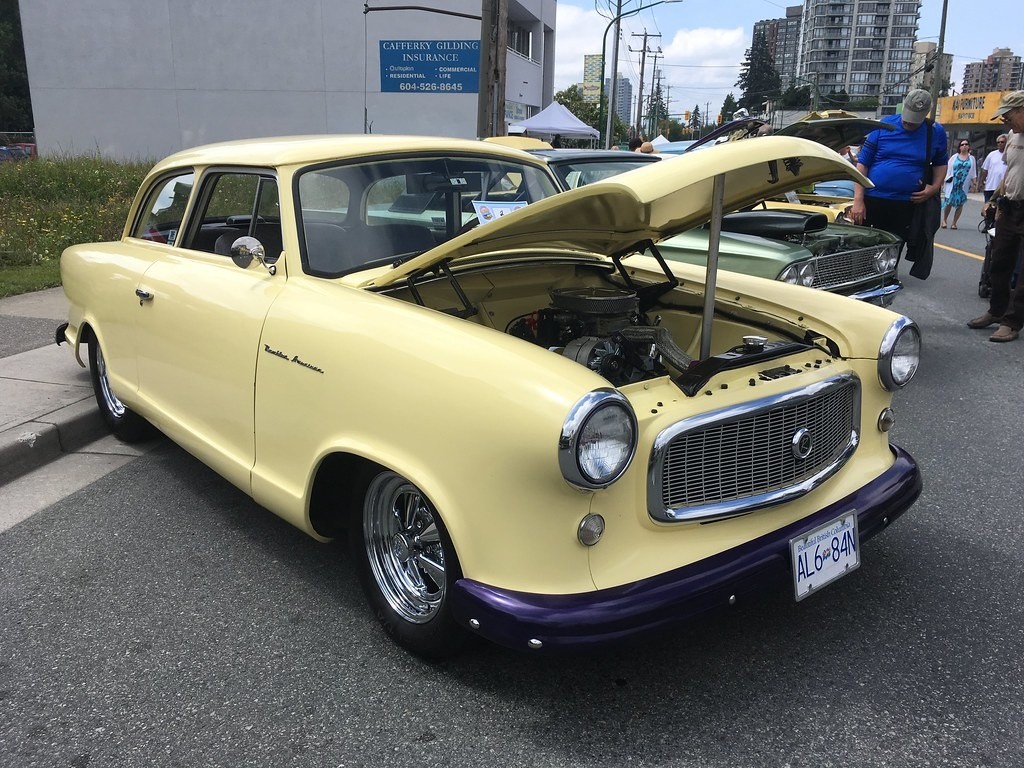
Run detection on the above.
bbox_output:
[902,89,932,123]
[757,124,774,136]
[990,90,1024,120]
[555,134,561,138]
[629,138,644,149]
[635,142,659,154]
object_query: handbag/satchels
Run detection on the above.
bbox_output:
[933,197,941,235]
[946,177,953,183]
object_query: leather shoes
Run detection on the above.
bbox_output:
[990,326,1020,342]
[967,312,1005,329]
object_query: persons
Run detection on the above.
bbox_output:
[977,134,1008,234]
[967,91,1024,342]
[549,132,564,150]
[726,106,774,147]
[606,137,666,155]
[839,144,864,167]
[942,139,974,230]
[850,90,949,291]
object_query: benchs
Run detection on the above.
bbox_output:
[192,216,364,254]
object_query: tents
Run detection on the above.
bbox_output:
[508,100,601,152]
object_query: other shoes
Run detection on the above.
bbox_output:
[982,225,991,233]
[941,222,947,228]
[951,225,957,229]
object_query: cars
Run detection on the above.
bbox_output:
[53,131,924,662]
[334,114,906,318]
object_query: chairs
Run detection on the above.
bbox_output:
[212,221,363,272]
[344,224,435,262]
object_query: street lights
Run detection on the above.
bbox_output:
[597,0,681,149]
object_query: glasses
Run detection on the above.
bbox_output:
[961,144,968,146]
[997,141,1004,143]
[902,121,914,126]
[1000,107,1023,123]
